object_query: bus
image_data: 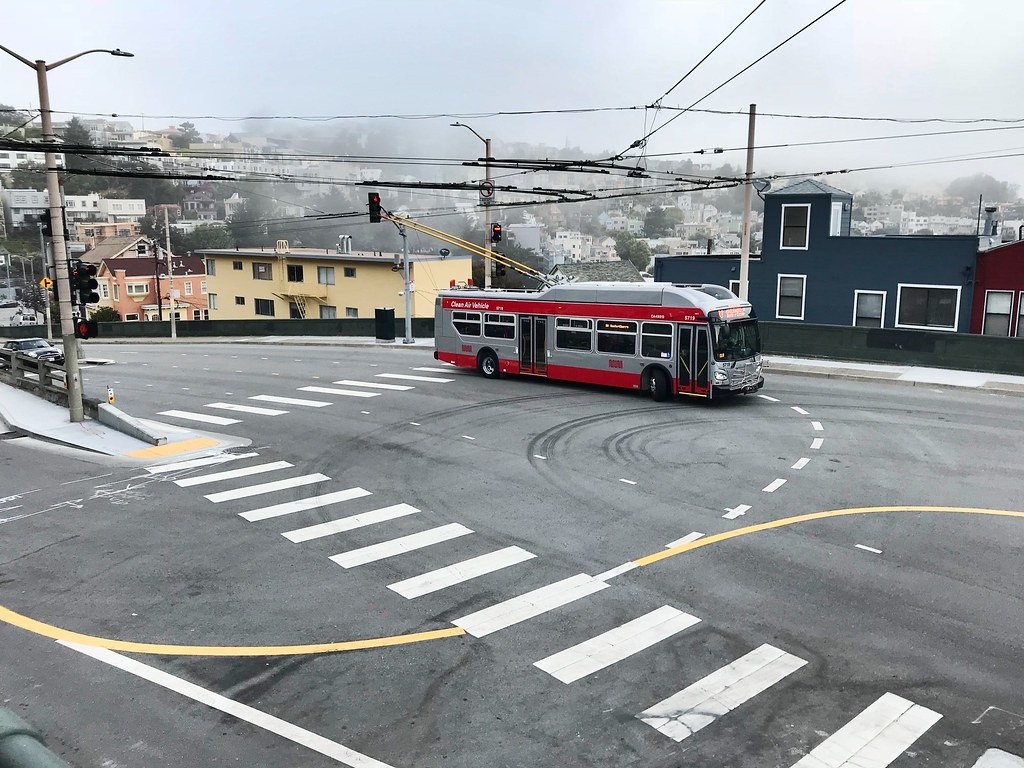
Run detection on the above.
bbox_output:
[434,282,764,403]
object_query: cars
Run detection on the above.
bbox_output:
[10,312,36,326]
[3,338,65,369]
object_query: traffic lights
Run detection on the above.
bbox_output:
[74,317,99,339]
[496,253,506,277]
[368,192,381,223]
[493,225,501,241]
[76,262,99,303]
[40,209,52,237]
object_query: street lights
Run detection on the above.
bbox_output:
[36,49,134,425]
[450,122,491,289]
[39,223,55,339]
